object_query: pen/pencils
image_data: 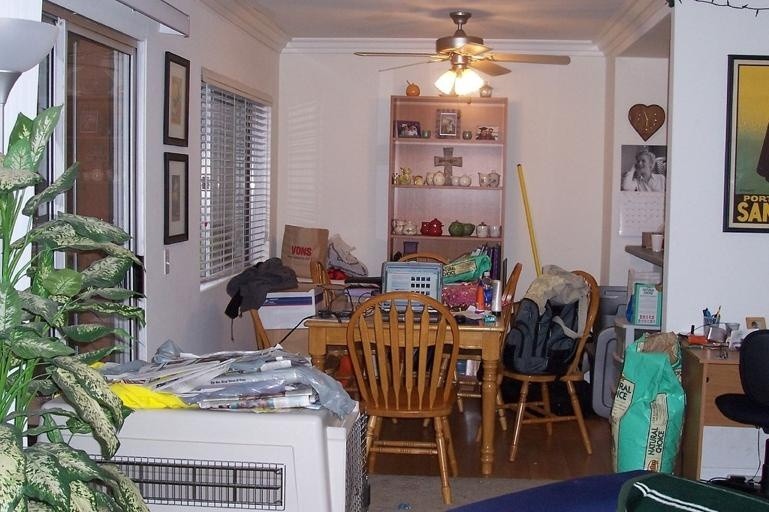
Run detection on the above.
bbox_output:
[703,305,722,323]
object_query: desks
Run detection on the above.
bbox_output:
[625,246,664,269]
[681,341,762,481]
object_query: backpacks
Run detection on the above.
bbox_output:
[503,265,588,375]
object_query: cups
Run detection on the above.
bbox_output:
[476,222,488,237]
[725,323,739,337]
[651,235,663,252]
[463,130,472,140]
[488,225,500,238]
[422,129,431,138]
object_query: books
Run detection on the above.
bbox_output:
[105,344,324,409]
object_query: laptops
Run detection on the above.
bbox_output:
[380,261,446,323]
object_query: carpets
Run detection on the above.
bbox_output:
[365,474,564,512]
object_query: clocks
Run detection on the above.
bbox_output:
[628,104,665,142]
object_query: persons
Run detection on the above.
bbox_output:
[622,150,666,192]
[752,322,759,328]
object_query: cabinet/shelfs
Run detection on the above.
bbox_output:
[387,95,509,280]
[609,319,661,395]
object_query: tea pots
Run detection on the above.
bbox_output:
[406,82,420,96]
[478,172,493,186]
[426,217,444,236]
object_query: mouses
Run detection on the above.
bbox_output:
[455,315,466,323]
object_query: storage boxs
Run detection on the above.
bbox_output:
[631,284,662,326]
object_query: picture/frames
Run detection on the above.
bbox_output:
[394,119,421,139]
[746,317,765,329]
[163,51,191,148]
[164,152,190,245]
[435,108,461,141]
[722,55,769,233]
[472,123,502,142]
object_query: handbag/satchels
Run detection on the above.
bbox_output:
[281,225,329,279]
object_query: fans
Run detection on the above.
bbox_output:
[354,11,571,77]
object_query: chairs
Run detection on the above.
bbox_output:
[715,329,769,496]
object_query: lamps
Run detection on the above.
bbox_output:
[434,55,486,97]
[0,18,61,156]
